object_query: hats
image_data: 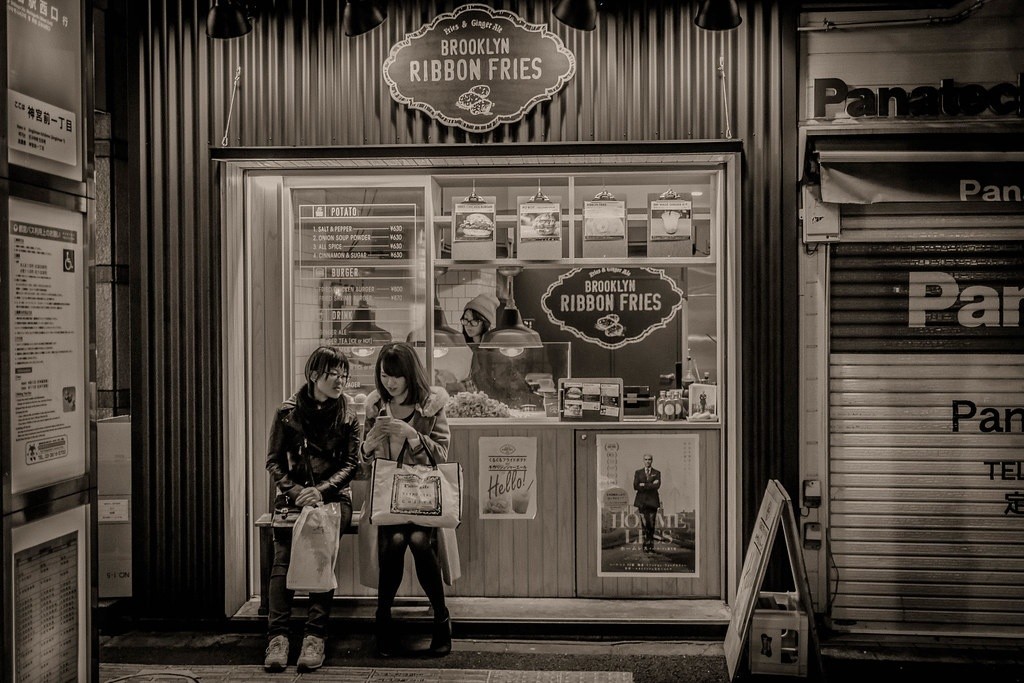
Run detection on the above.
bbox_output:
[464,293,500,324]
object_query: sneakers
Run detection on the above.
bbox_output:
[264,635,290,667]
[297,635,326,668]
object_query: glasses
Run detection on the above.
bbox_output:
[460,318,482,327]
[324,370,351,381]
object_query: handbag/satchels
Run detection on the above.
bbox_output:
[271,502,341,593]
[369,432,463,529]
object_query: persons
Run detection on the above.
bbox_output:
[633,453,662,552]
[361,342,463,658]
[263,346,360,672]
[446,293,501,396]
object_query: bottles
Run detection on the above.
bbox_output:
[657,391,667,419]
[674,391,683,420]
[663,391,675,421]
[704,372,710,383]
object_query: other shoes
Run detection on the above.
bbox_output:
[375,609,399,657]
[430,607,452,655]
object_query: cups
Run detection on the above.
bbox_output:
[512,500,529,514]
[661,212,680,234]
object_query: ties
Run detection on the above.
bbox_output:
[646,469,649,481]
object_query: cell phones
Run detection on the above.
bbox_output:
[376,416,391,437]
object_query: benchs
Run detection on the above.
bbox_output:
[254,461,441,615]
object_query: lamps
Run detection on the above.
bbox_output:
[344,0,388,38]
[406,267,467,358]
[479,266,543,357]
[329,268,391,357]
[694,0,744,31]
[205,0,253,40]
[552,0,597,31]
